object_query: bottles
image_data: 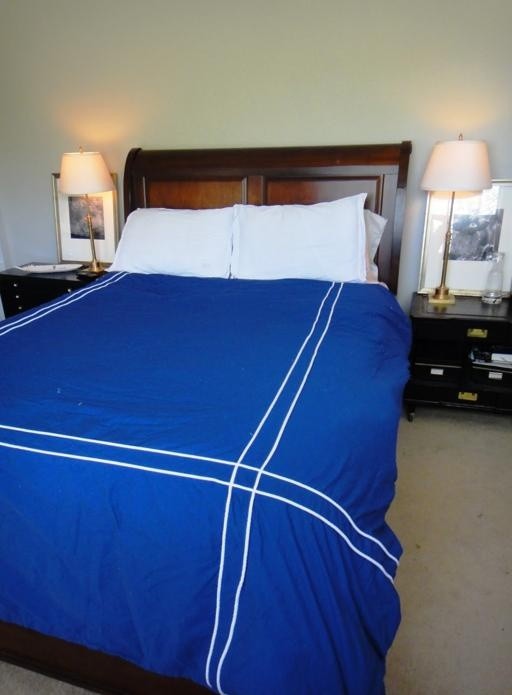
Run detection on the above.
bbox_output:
[482,253,504,305]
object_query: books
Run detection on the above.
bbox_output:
[473,349,512,362]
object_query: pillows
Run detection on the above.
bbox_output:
[102,190,390,285]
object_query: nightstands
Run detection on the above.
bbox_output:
[404,292,512,422]
[1,261,113,320]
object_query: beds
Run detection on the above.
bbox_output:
[0,139,412,695]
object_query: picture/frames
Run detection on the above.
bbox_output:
[417,179,512,298]
[51,172,118,267]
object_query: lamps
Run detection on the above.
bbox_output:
[56,146,116,278]
[419,132,492,305]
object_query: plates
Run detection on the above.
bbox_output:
[15,263,85,275]
